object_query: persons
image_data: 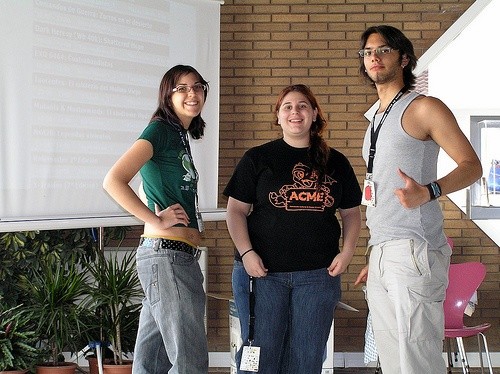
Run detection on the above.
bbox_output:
[223,84,362,374]
[355,24,483,374]
[101,63,213,374]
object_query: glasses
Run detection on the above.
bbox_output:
[358,47,400,58]
[173,82,207,93]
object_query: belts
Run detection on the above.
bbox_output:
[139,236,202,261]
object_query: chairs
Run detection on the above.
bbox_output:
[445,262,493,374]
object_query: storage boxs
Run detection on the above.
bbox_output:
[205,292,360,374]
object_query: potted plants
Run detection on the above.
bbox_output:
[0,225,145,374]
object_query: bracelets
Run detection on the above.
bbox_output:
[240,249,252,258]
[427,180,443,202]
